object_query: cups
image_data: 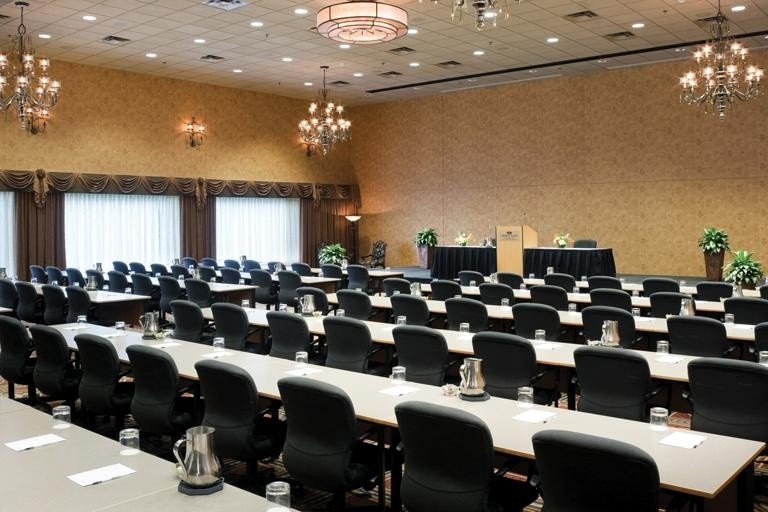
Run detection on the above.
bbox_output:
[658,341,669,356]
[52,405,72,429]
[76,316,87,325]
[398,316,406,324]
[649,407,669,430]
[459,323,469,335]
[264,481,291,512]
[589,340,600,347]
[294,351,309,367]
[241,300,249,308]
[268,255,768,324]
[115,322,125,334]
[760,350,768,366]
[392,367,406,385]
[535,329,546,342]
[118,427,140,456]
[518,387,534,406]
[280,304,288,311]
[213,337,224,351]
[337,309,344,316]
[485,237,493,248]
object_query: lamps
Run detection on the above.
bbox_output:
[419,0,526,31]
[679,0,763,122]
[298,65,352,157]
[0,1,61,131]
[316,0,408,44]
[185,117,205,147]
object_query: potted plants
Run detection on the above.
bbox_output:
[415,228,438,269]
[698,226,731,281]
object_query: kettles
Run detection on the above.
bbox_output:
[299,293,315,315]
[174,424,224,492]
[600,320,620,347]
[461,358,487,396]
[139,312,159,337]
